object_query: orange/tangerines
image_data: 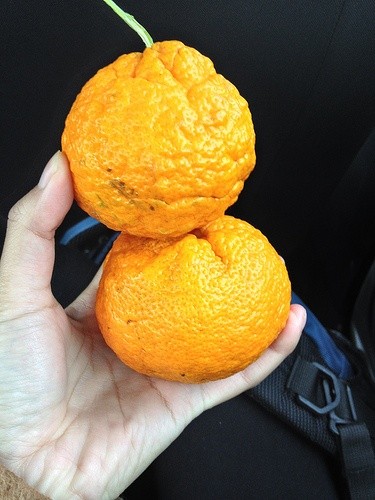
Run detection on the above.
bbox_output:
[96,214,290,382]
[59,0,256,239]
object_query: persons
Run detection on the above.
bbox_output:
[0,150,307,500]
